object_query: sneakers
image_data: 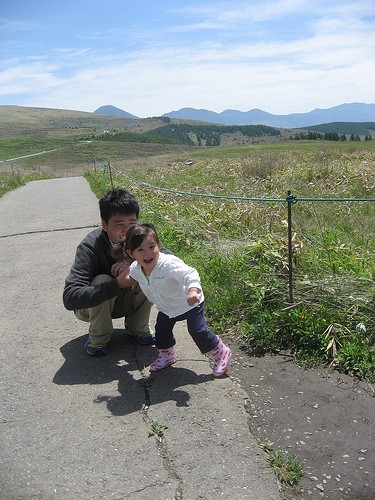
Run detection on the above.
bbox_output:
[86,335,111,355]
[125,328,156,345]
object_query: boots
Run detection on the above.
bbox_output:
[148,346,178,371]
[210,335,231,377]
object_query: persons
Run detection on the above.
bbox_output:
[63,186,156,356]
[121,222,231,377]
